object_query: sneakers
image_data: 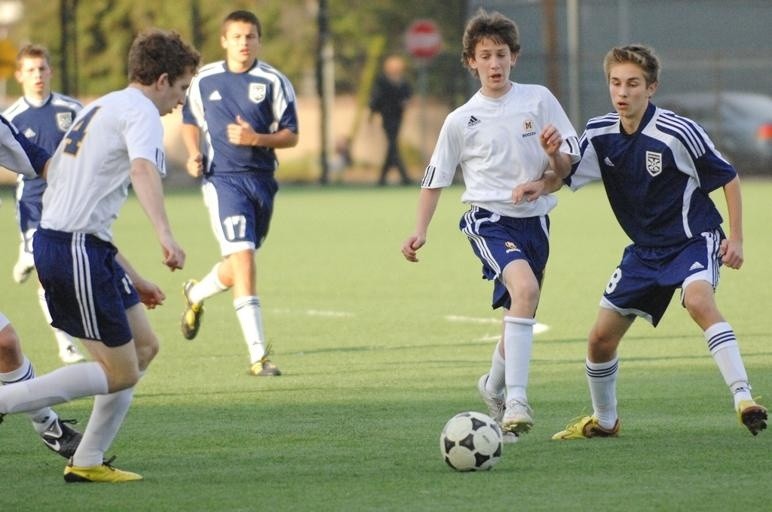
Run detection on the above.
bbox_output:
[478,374,506,424]
[13,244,36,283]
[64,464,143,483]
[39,417,85,459]
[738,401,768,435]
[182,279,203,340]
[59,348,85,364]
[552,416,620,440]
[501,400,534,432]
[247,360,279,376]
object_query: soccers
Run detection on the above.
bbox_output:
[440,412,503,470]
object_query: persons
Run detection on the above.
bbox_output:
[511,42,766,440]
[0,44,85,364]
[179,9,301,377]
[0,27,201,482]
[0,112,85,459]
[400,9,583,443]
[365,54,418,183]
